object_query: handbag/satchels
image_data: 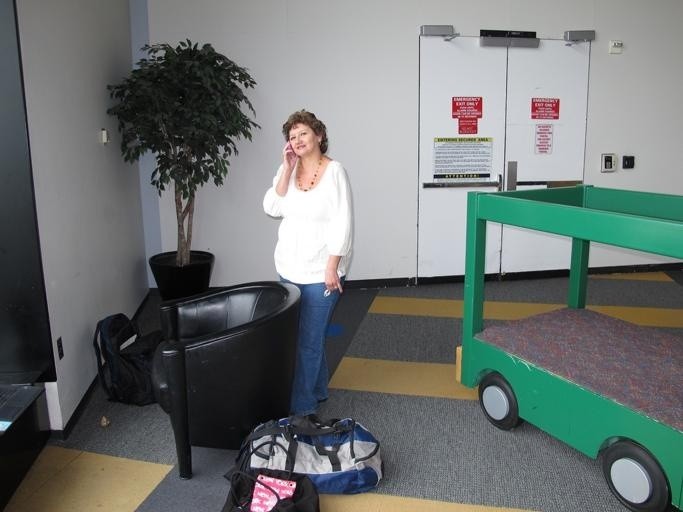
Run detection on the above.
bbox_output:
[222,416,384,512]
[93,313,161,407]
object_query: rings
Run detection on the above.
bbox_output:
[334,286,337,289]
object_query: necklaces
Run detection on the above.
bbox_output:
[297,153,324,192]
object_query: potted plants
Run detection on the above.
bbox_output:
[105,35,261,299]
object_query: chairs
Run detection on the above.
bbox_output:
[147,279,302,481]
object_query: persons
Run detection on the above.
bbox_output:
[263,109,355,423]
